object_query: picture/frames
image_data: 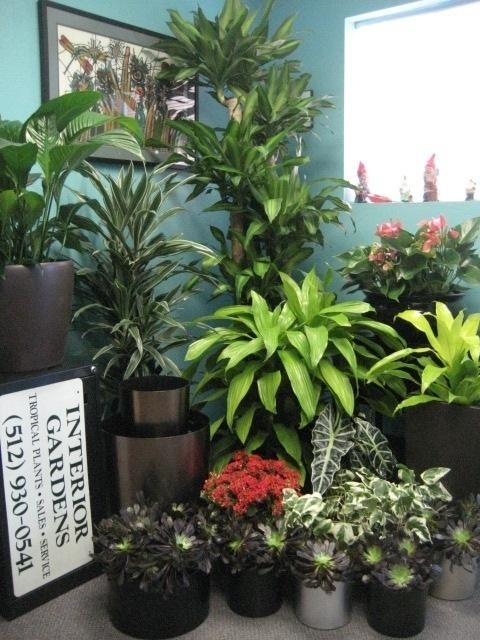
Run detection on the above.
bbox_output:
[31,1,207,173]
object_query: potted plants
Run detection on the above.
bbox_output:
[192,430,480,638]
[0,83,148,371]
[363,302,480,490]
[90,491,213,638]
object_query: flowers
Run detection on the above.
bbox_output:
[332,210,480,287]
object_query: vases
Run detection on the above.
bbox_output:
[354,287,471,356]
